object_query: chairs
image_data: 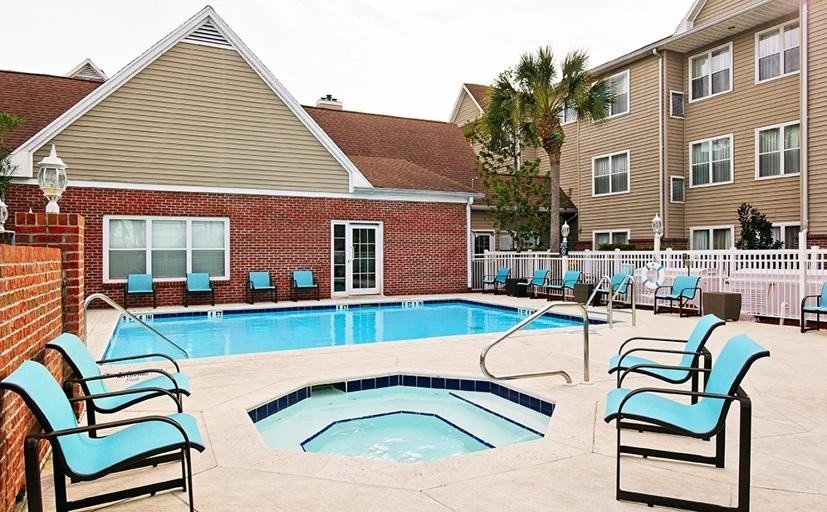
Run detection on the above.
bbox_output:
[245,271,277,303]
[604,313,770,512]
[185,273,214,307]
[483,268,702,318]
[0,331,206,512]
[123,273,156,308]
[801,282,827,333]
[290,270,320,302]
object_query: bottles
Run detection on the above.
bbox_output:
[723,277,729,291]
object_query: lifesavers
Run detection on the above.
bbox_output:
[641,262,665,289]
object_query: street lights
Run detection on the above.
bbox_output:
[650,211,663,252]
[35,142,75,213]
[561,220,572,246]
